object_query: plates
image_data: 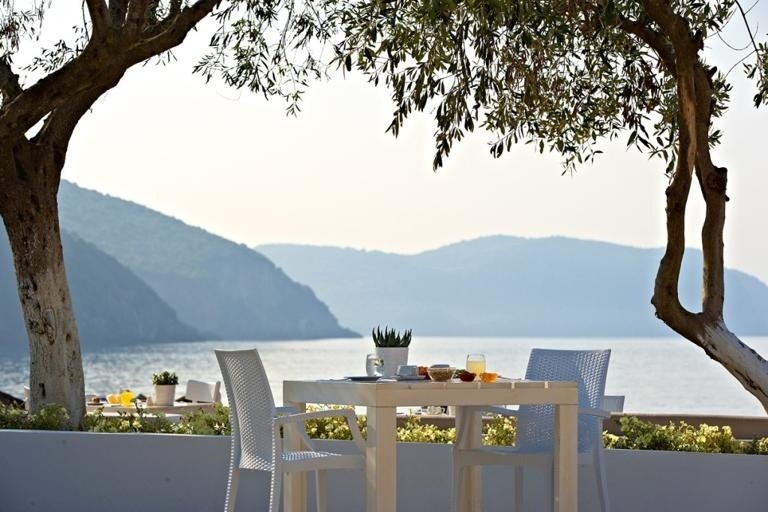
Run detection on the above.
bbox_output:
[391,375,426,381]
[344,375,382,381]
[87,402,105,406]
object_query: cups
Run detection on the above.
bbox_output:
[398,365,419,376]
[466,353,486,381]
[418,366,424,376]
[366,353,382,376]
[91,392,132,406]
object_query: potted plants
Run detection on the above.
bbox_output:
[150,372,178,405]
[373,325,412,377]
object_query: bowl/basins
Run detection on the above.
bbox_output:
[458,372,476,381]
[427,368,457,382]
[479,372,498,382]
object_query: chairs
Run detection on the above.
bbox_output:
[452,349,611,509]
[22,386,31,413]
[214,348,365,511]
[185,379,221,404]
